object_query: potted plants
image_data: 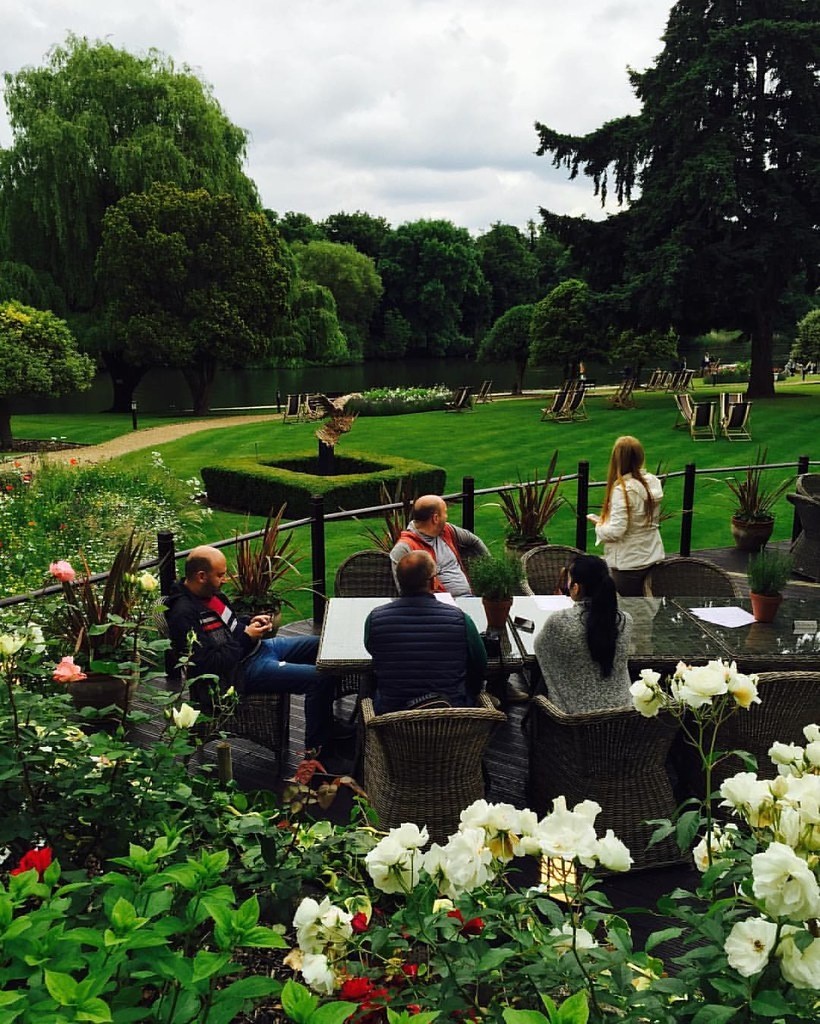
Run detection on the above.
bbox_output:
[468,552,528,628]
[221,502,329,639]
[747,544,794,622]
[46,528,172,736]
[725,446,797,549]
[480,448,577,559]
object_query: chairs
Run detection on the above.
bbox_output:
[605,376,638,411]
[674,393,753,442]
[474,380,493,403]
[541,379,592,424]
[284,394,342,424]
[786,473,820,585]
[445,385,474,411]
[335,544,820,878]
[154,596,290,781]
[638,370,696,394]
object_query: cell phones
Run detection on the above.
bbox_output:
[587,513,600,523]
[262,614,275,627]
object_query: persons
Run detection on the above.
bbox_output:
[364,548,487,716]
[708,357,719,387]
[389,495,530,703]
[587,436,668,598]
[534,556,634,716]
[163,544,352,777]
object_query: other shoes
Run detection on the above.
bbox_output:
[327,765,353,776]
[487,693,501,706]
[328,722,353,739]
[486,680,528,702]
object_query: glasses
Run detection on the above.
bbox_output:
[429,567,442,580]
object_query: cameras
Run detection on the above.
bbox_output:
[513,617,535,634]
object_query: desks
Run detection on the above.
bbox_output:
[316,594,820,725]
